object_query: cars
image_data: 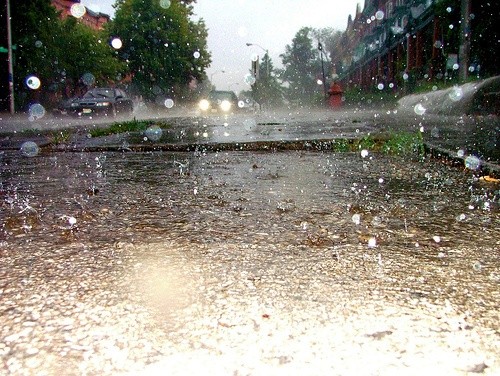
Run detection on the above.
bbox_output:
[202,90,239,114]
[78,89,135,118]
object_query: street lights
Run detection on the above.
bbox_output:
[210,70,226,90]
[246,42,271,111]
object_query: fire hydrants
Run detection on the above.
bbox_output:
[326,83,343,110]
